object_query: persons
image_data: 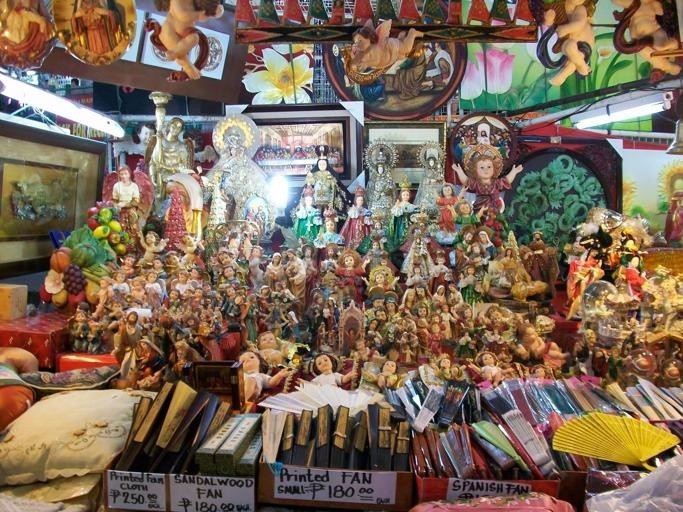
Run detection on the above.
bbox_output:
[39,117,683,401]
[542,0,595,86]
[349,18,423,71]
[609,0,682,76]
[158,0,224,81]
[421,41,454,89]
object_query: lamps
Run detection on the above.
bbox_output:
[0,71,125,139]
[570,92,673,131]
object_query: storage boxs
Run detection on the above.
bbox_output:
[413,459,561,503]
[103,459,257,512]
[258,452,413,511]
[561,470,587,512]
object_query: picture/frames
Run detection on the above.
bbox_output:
[225,101,364,191]
[106,114,226,173]
[363,120,447,191]
[0,119,106,280]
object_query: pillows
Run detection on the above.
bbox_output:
[0,388,160,486]
